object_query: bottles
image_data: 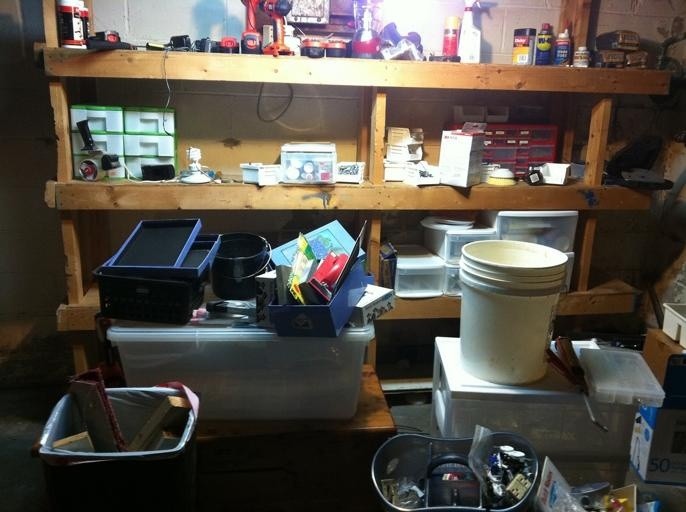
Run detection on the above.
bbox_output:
[511,27,536,65]
[555,33,572,66]
[442,16,459,56]
[572,47,590,68]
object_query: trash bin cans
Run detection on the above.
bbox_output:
[39,387,199,512]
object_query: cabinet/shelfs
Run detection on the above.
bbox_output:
[31,41,683,396]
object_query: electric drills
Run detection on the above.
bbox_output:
[258,0,291,55]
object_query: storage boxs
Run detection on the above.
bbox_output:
[107,319,375,418]
[349,283,395,329]
[96,262,204,325]
[270,258,368,336]
[69,101,179,181]
[280,142,339,184]
[385,121,572,191]
[624,467,684,510]
[627,396,683,484]
[380,211,577,297]
[434,336,638,457]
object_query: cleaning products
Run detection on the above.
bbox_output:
[457,0,483,64]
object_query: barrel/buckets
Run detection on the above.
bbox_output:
[211,232,270,300]
[460,239,569,387]
